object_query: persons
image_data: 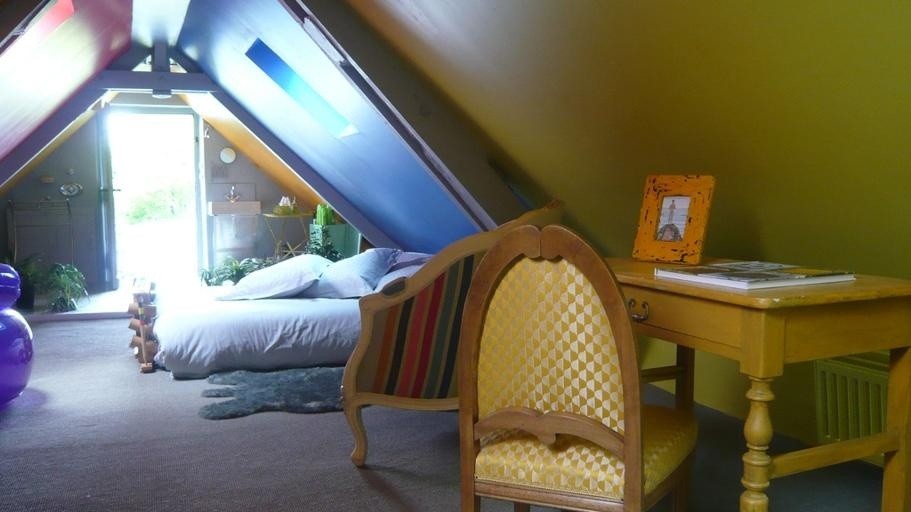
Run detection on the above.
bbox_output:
[668,199,677,225]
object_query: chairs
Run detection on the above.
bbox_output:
[455,223,702,511]
[342,197,567,468]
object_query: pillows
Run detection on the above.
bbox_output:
[216,246,402,299]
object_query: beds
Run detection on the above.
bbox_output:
[126,250,437,378]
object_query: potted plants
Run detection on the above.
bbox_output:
[308,203,362,262]
[17,251,90,313]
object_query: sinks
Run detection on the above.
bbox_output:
[208,201,262,215]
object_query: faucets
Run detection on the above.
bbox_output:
[229,185,237,201]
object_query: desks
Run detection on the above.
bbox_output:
[263,211,314,259]
[599,253,911,512]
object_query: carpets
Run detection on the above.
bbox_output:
[199,365,348,420]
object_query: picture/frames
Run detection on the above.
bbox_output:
[632,173,717,267]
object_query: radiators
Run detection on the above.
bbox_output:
[809,351,890,469]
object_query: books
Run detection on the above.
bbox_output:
[654,260,856,291]
